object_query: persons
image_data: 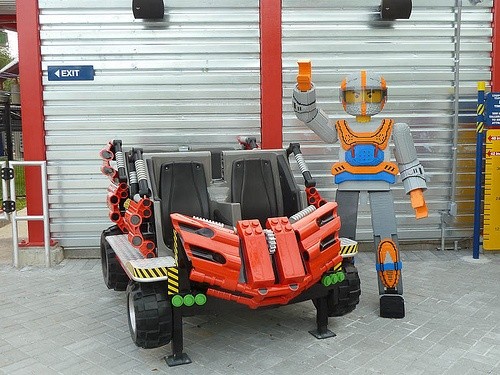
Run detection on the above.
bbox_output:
[293,59,430,319]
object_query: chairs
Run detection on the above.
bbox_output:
[160,160,214,252]
[229,156,288,230]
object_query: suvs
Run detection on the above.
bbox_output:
[92,136,361,349]
